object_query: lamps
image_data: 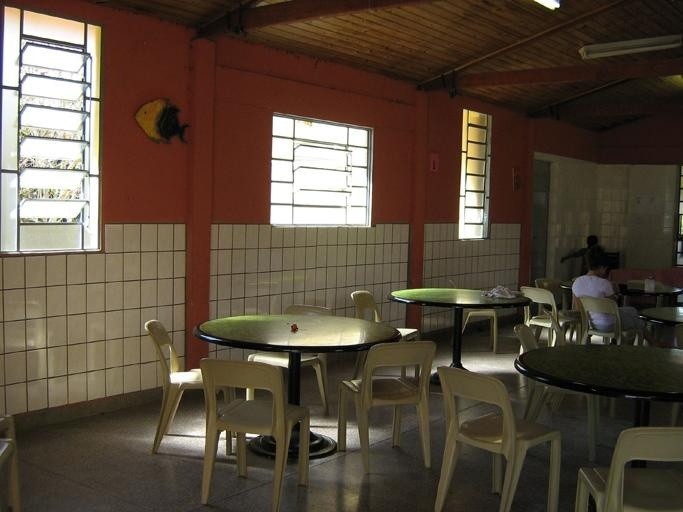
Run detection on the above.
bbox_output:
[576,35,683,60]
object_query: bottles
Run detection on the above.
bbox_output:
[644,277,655,293]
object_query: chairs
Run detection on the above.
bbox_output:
[571,425,681,512]
[514,324,599,464]
[243,302,331,413]
[199,357,313,512]
[336,337,435,466]
[432,365,566,512]
[141,316,233,462]
[349,289,419,383]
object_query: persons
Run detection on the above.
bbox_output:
[572,245,653,345]
[560,235,598,273]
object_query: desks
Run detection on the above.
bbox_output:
[513,341,682,470]
[196,313,402,460]
